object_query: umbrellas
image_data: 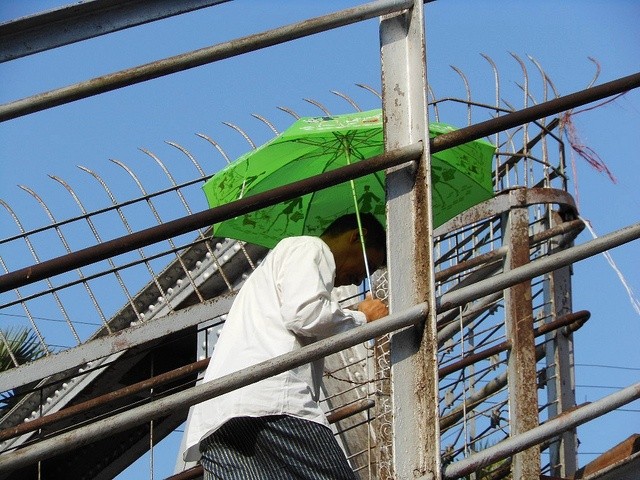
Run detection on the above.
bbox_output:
[201,107,497,302]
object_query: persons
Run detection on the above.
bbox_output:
[182,212,388,480]
